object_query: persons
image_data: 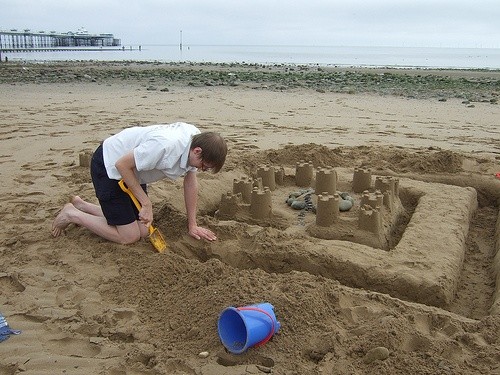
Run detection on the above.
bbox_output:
[52,122,227,245]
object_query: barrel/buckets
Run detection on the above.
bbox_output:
[218,302,280,354]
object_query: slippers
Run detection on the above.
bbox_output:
[0,313,11,335]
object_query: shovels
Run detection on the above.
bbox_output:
[117,179,168,253]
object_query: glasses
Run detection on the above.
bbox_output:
[200,158,207,172]
